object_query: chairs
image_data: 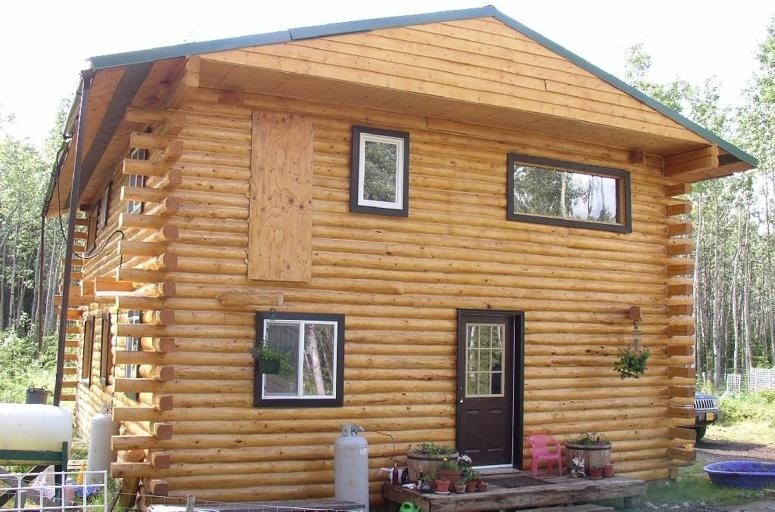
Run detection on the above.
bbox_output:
[528,433,564,478]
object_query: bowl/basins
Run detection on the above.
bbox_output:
[701,460,775,490]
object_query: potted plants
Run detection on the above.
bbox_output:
[251,344,293,374]
[563,440,615,480]
[407,442,488,495]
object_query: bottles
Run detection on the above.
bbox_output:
[392,461,399,485]
